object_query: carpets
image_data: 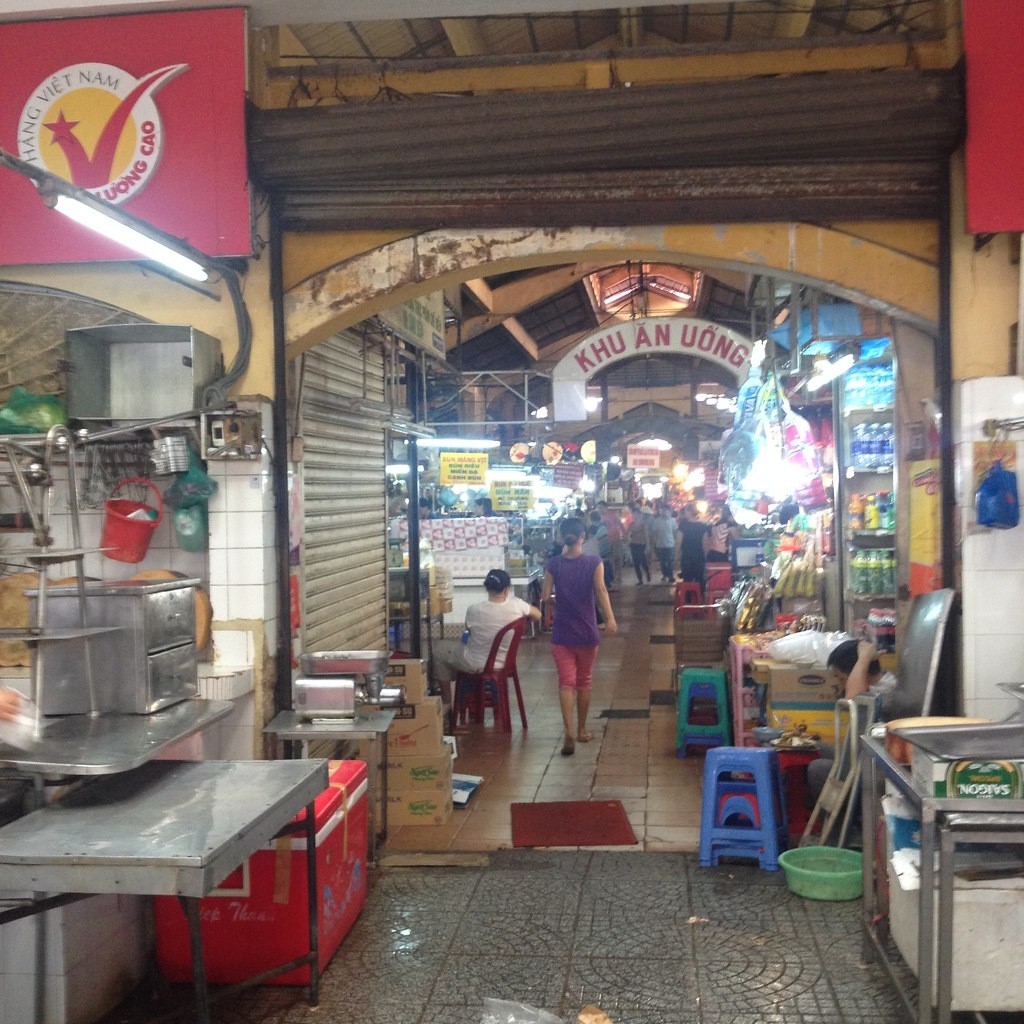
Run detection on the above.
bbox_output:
[510,799,639,847]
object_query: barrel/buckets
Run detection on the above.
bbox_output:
[98,478,162,563]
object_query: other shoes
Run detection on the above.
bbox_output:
[801,833,845,847]
[560,743,575,754]
[578,732,592,741]
[844,824,862,848]
[668,578,675,583]
[661,577,665,582]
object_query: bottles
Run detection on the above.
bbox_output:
[850,423,894,468]
[842,361,893,406]
[863,608,896,647]
[850,549,897,595]
[847,490,895,531]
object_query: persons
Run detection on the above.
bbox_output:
[474,498,497,517]
[549,497,734,603]
[808,639,898,847]
[433,569,542,729]
[540,517,617,754]
[419,498,438,519]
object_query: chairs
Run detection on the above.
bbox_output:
[452,616,530,734]
[675,582,731,621]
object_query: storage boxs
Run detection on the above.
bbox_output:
[153,760,368,986]
[703,562,731,590]
[750,658,851,747]
[354,659,453,826]
[886,742,1024,1014]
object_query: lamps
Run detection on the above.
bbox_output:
[37,178,208,282]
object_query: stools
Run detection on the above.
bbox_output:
[537,592,556,636]
[674,668,820,871]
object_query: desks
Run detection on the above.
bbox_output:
[276,731,388,868]
[0,760,330,1024]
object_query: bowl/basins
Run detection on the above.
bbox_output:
[778,845,863,901]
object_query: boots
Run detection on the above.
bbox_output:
[634,569,643,586]
[645,568,651,581]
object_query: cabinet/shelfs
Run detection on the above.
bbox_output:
[836,376,895,636]
[728,635,771,748]
[23,576,204,715]
